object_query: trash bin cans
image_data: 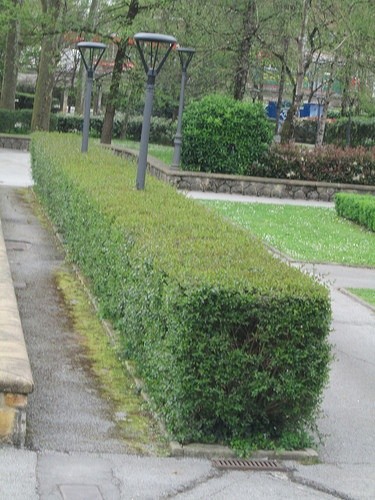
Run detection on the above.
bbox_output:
[266,99,296,121]
[300,102,325,119]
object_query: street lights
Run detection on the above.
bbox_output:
[76,40,107,153]
[171,46,195,170]
[133,30,179,189]
